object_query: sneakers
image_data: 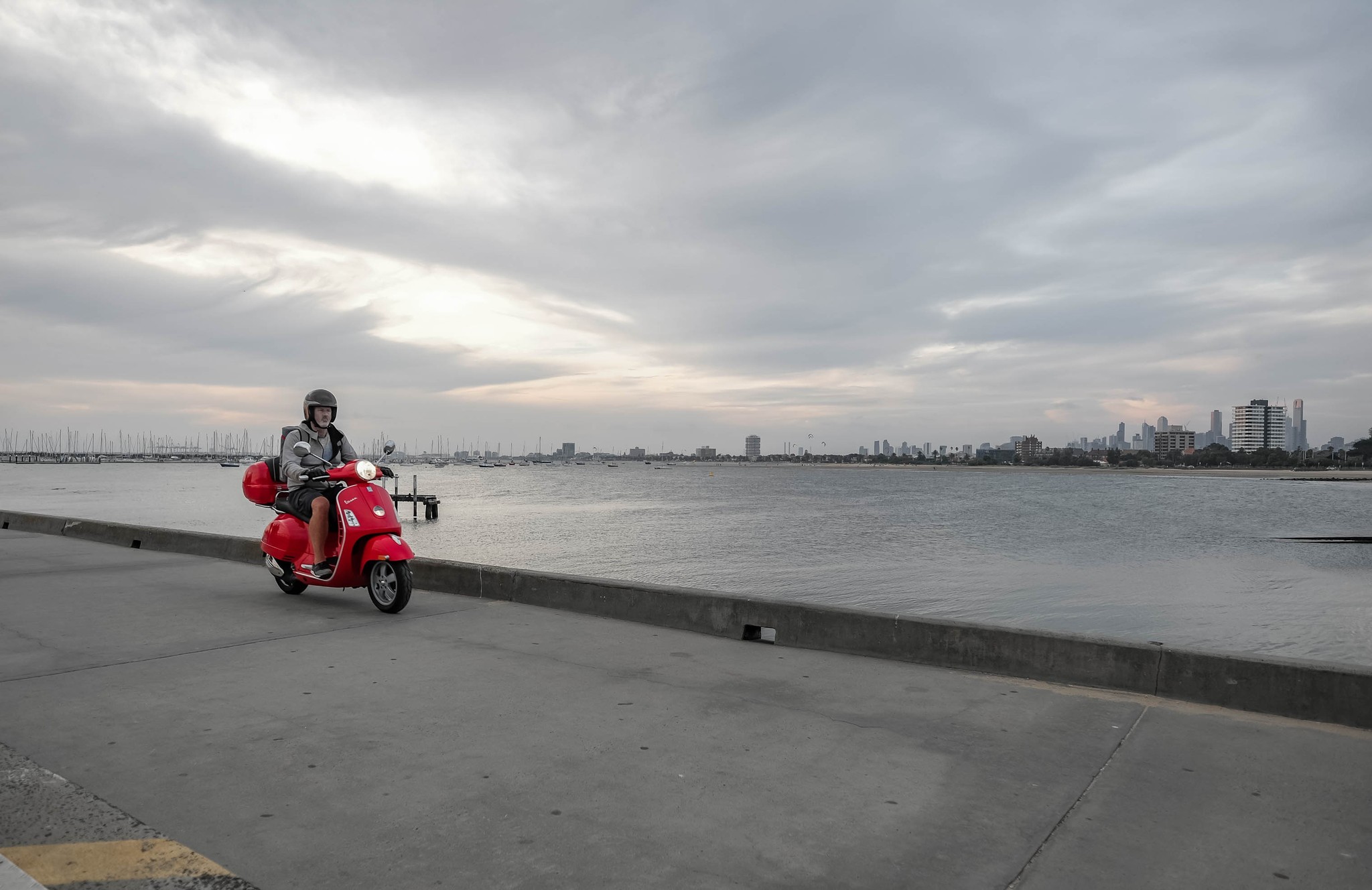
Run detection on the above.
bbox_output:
[310,561,333,579]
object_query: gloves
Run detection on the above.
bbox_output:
[301,468,329,483]
[377,466,394,480]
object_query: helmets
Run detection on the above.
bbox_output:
[303,389,337,429]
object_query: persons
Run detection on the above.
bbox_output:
[282,389,394,580]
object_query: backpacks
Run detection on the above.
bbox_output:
[279,426,303,483]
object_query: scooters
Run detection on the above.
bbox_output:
[242,441,414,614]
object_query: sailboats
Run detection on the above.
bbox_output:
[96,428,677,470]
